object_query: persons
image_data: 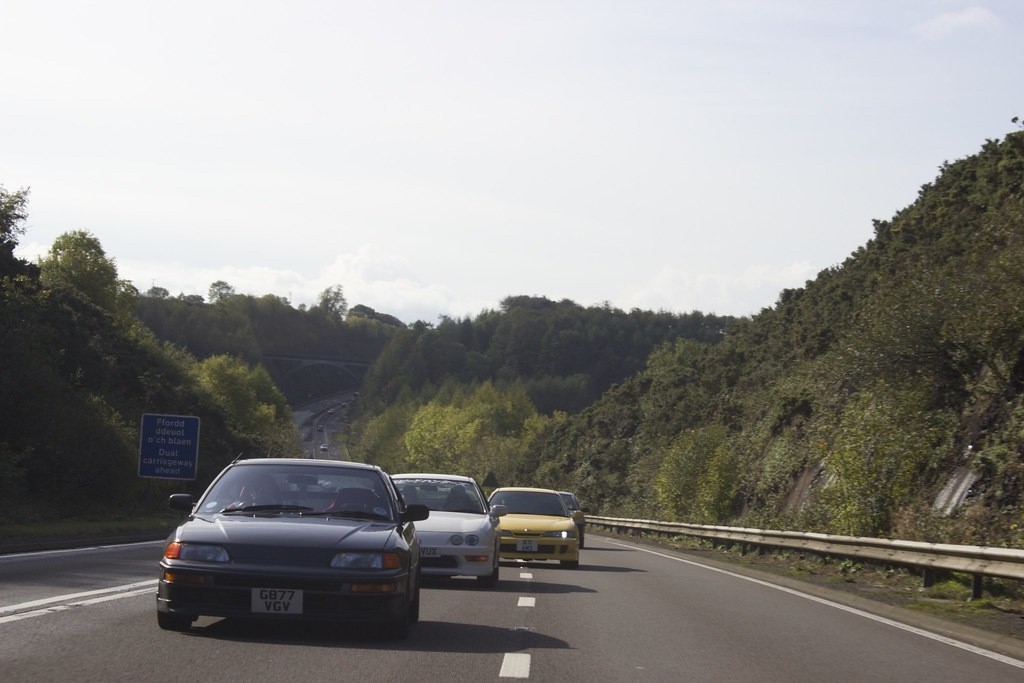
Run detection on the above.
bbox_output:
[220,476,281,513]
[502,495,513,512]
[451,485,469,509]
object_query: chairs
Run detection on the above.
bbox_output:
[401,484,419,505]
[225,481,278,510]
[444,485,472,512]
[324,487,383,514]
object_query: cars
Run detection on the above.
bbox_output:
[317,425,325,432]
[320,444,328,450]
[155,457,429,638]
[328,408,335,414]
[557,491,589,550]
[372,473,507,588]
[484,487,584,569]
